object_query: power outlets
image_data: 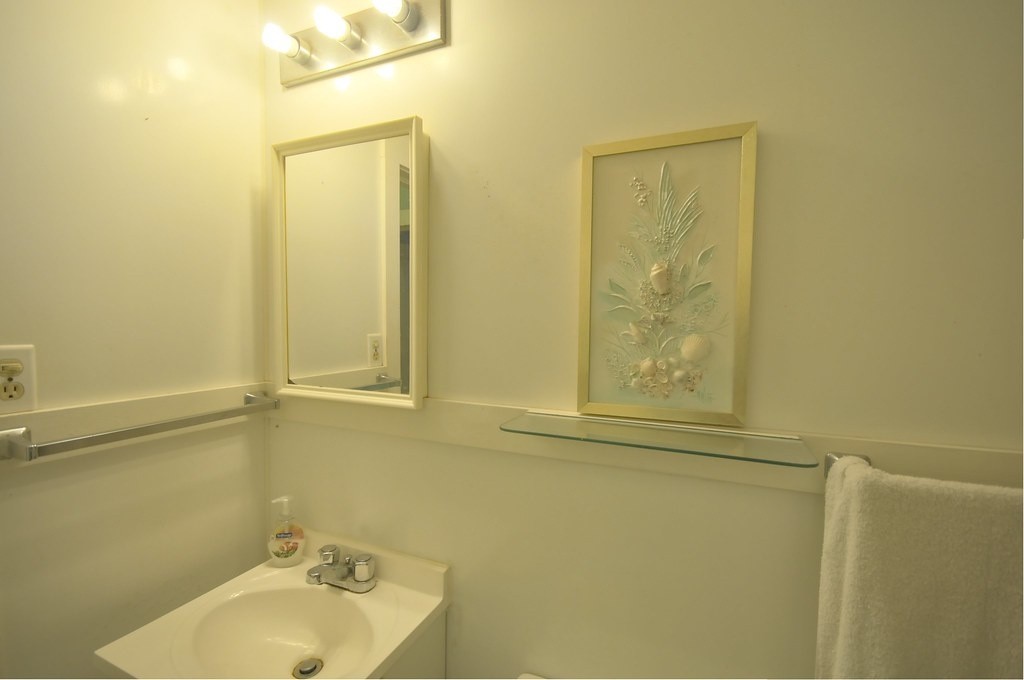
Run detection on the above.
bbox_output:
[1,345,37,415]
[367,334,382,367]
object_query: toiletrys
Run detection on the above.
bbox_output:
[267,495,308,566]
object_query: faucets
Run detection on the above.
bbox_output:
[307,562,352,585]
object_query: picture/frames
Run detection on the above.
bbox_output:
[576,119,757,426]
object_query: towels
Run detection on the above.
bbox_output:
[812,455,1022,678]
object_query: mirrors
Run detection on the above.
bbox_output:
[274,111,424,413]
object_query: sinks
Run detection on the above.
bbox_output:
[193,585,372,679]
